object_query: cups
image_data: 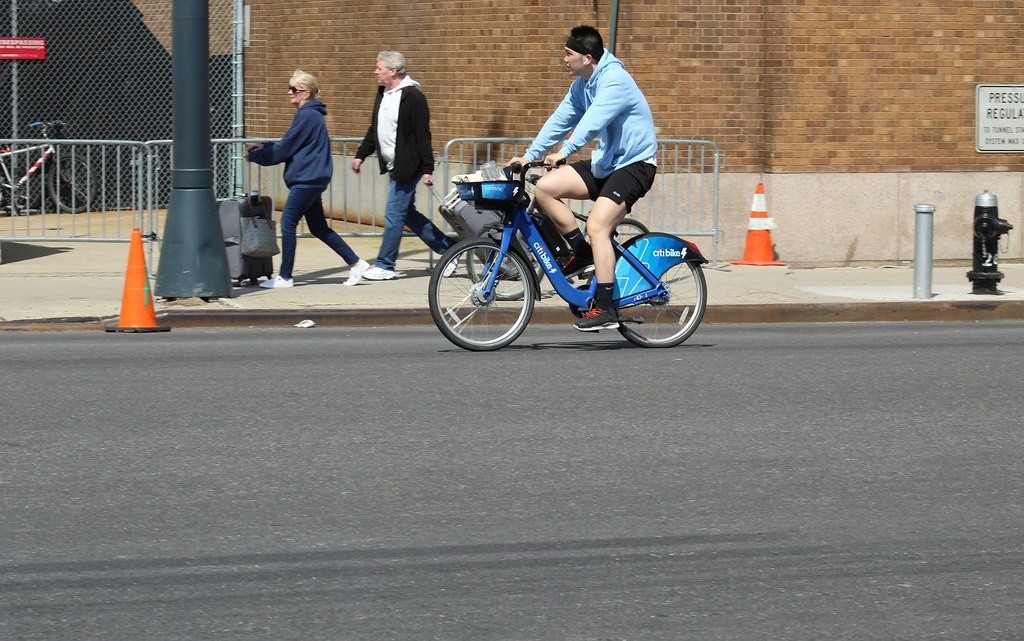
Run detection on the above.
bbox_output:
[480,160,501,181]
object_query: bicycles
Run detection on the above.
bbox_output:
[466,174,650,302]
[428,161,710,349]
[0,122,99,216]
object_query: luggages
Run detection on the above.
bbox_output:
[425,180,536,280]
[217,161,273,286]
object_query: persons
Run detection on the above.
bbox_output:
[243,69,370,287]
[352,51,463,281]
[506,25,658,332]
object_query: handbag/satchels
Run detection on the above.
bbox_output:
[240,198,280,258]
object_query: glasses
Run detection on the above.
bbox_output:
[288,85,307,93]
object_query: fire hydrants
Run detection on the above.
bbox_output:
[965,191,1014,296]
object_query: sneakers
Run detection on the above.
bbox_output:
[572,306,620,331]
[362,267,397,280]
[343,260,370,286]
[562,242,595,279]
[259,276,294,289]
[442,254,461,278]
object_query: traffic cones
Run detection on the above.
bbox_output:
[105,226,171,333]
[728,182,786,267]
